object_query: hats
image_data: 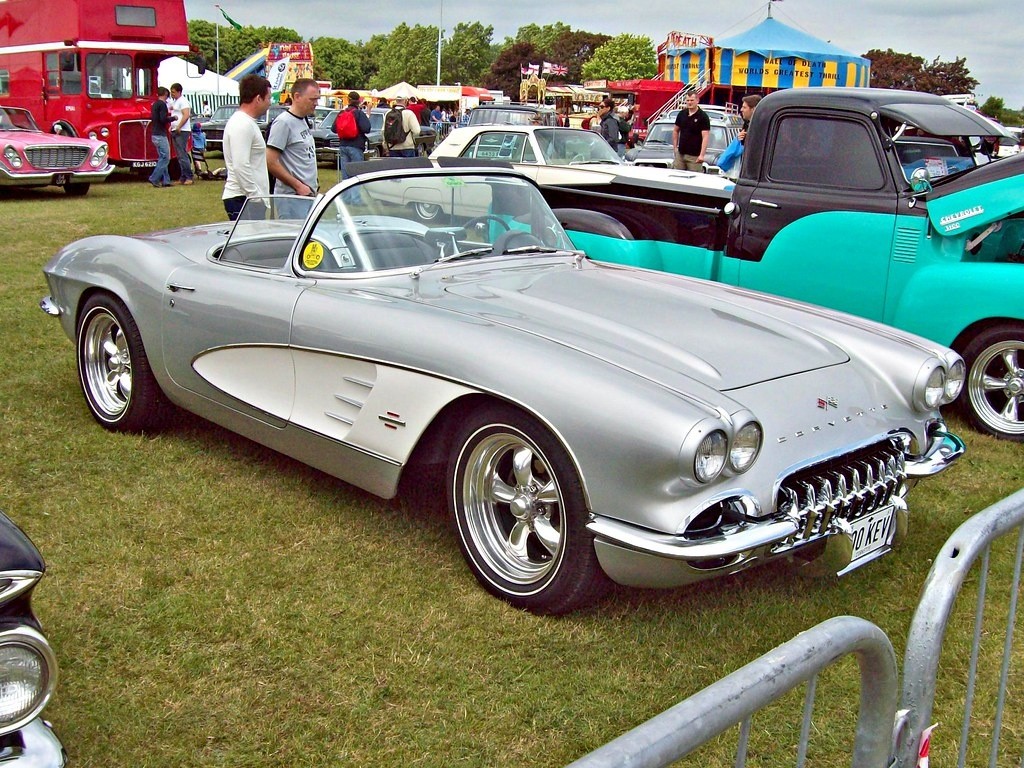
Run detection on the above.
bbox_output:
[395,93,408,101]
[192,122,202,131]
[348,92,360,100]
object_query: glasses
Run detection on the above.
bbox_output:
[599,105,606,109]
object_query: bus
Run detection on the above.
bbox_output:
[1,1,206,178]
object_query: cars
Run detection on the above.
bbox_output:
[0,106,116,196]
[42,167,965,623]
[186,96,749,184]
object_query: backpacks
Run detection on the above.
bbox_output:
[336,107,359,140]
[384,107,411,144]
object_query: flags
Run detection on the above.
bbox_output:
[543,61,568,76]
[520,67,534,75]
[529,64,540,75]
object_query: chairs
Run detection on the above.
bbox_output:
[213,236,338,272]
[344,232,439,274]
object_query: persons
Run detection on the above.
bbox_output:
[598,98,619,152]
[148,86,178,188]
[405,96,426,125]
[192,122,209,173]
[376,97,391,108]
[168,83,194,185]
[449,109,456,122]
[613,106,631,157]
[331,91,371,207]
[463,106,472,123]
[221,74,272,221]
[417,98,431,127]
[430,105,442,122]
[266,77,321,220]
[716,95,763,181]
[382,94,422,157]
[202,101,212,121]
[672,90,710,173]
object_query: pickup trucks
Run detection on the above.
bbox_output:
[475,85,1023,442]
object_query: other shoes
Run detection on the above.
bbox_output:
[148,178,160,188]
[161,184,174,187]
[174,180,194,185]
